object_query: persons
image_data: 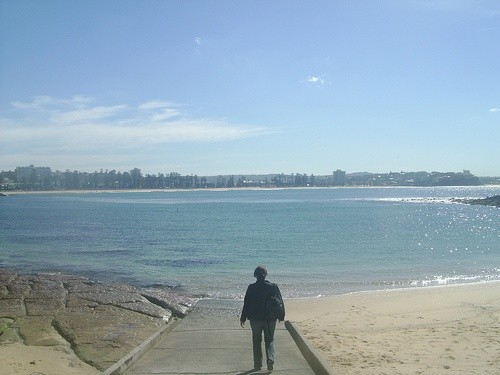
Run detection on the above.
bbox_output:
[240,265,286,370]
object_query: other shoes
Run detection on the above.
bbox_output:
[255,366,260,370]
[267,360,273,370]
